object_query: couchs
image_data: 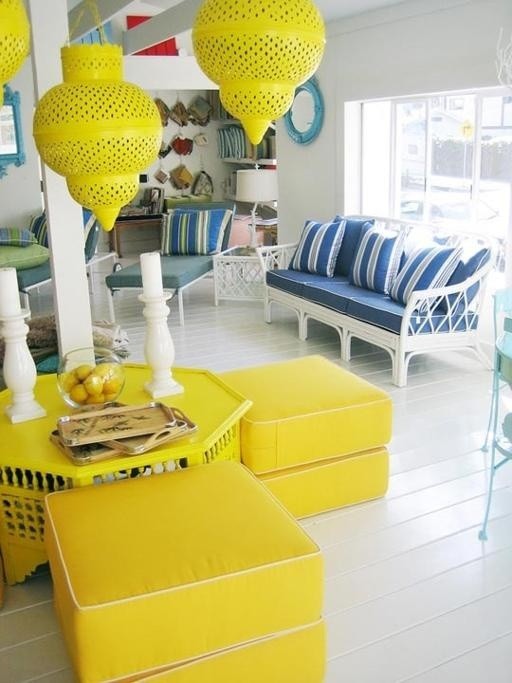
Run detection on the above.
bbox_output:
[257,212,498,387]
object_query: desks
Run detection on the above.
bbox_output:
[211,243,276,308]
[0,355,256,589]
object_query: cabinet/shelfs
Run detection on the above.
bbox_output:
[214,113,281,207]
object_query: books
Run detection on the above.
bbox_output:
[142,188,166,214]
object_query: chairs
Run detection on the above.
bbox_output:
[1,195,232,335]
[477,295,512,541]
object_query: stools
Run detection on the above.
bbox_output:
[41,456,327,683]
[218,354,396,523]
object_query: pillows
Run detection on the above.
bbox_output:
[288,215,490,314]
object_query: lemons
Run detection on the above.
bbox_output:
[63,362,121,407]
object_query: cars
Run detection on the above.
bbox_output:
[400,190,510,233]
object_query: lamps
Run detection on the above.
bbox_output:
[0,1,31,106]
[190,0,327,152]
[235,160,279,248]
[31,0,163,234]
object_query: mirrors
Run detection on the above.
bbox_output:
[284,72,324,146]
[0,83,26,178]
